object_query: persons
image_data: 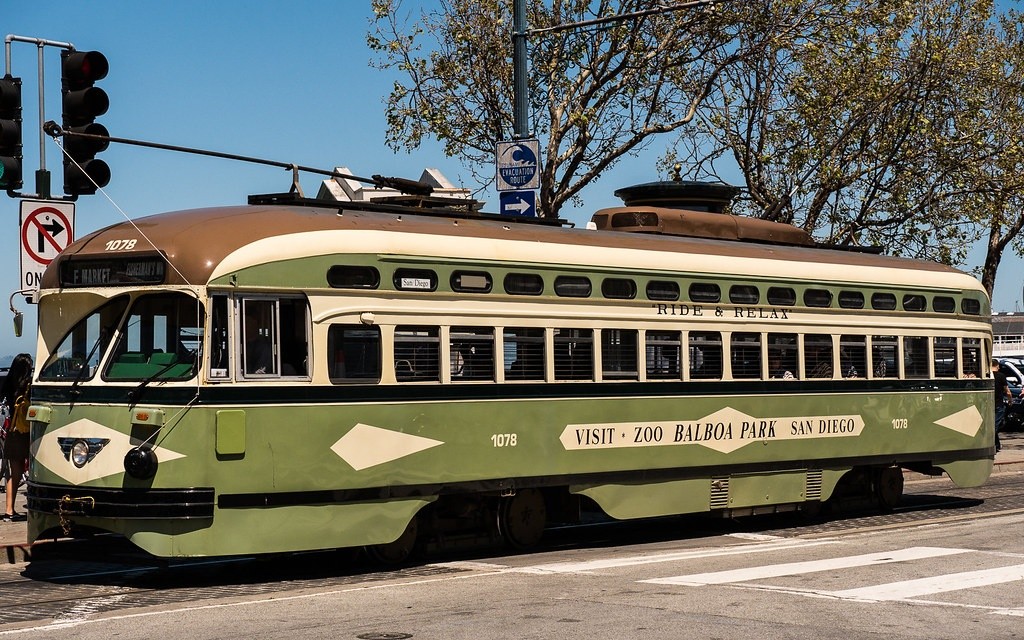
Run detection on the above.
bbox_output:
[555,335,581,380]
[904,338,938,377]
[243,318,286,374]
[0,353,33,522]
[992,359,1012,452]
[510,340,543,375]
[459,341,493,376]
[1019,385,1024,426]
[809,345,886,379]
[700,333,793,379]
[953,347,975,378]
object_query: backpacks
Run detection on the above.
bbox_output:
[10,382,31,432]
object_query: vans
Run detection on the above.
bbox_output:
[936,358,1024,414]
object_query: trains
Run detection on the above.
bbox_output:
[9,166,1000,571]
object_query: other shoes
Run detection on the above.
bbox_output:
[996,445,1001,452]
[3,512,27,521]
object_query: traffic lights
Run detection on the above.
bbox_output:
[60,49,111,194]
[0,73,24,191]
